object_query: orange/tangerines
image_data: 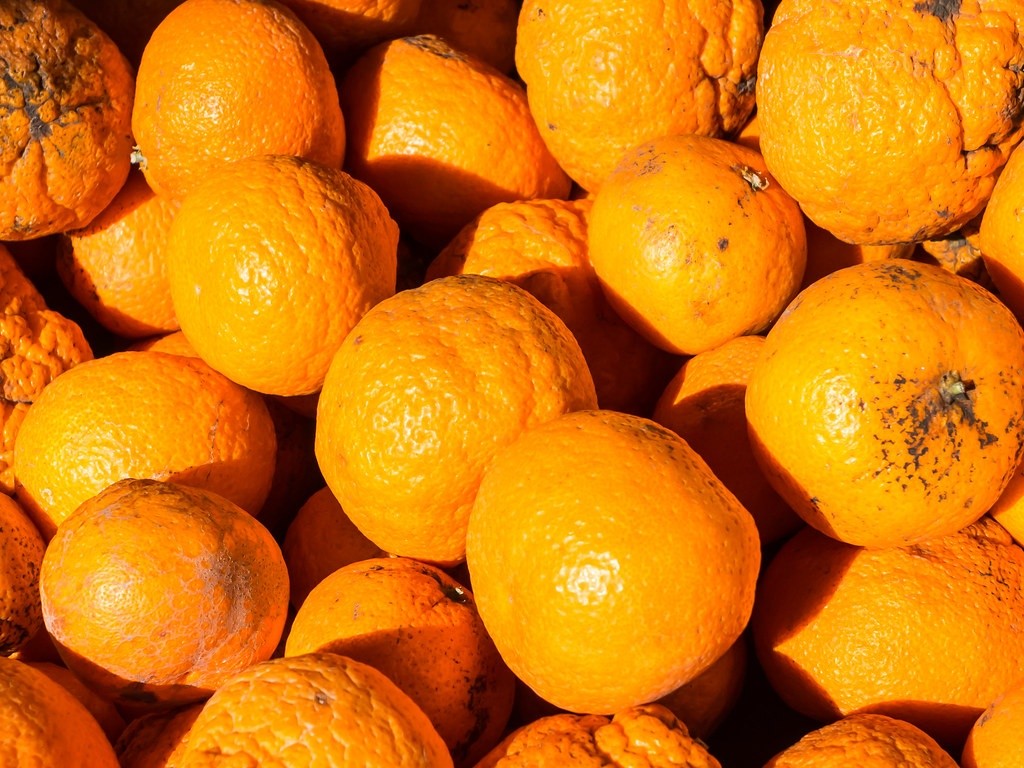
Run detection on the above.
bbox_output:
[1,0,1024,767]
